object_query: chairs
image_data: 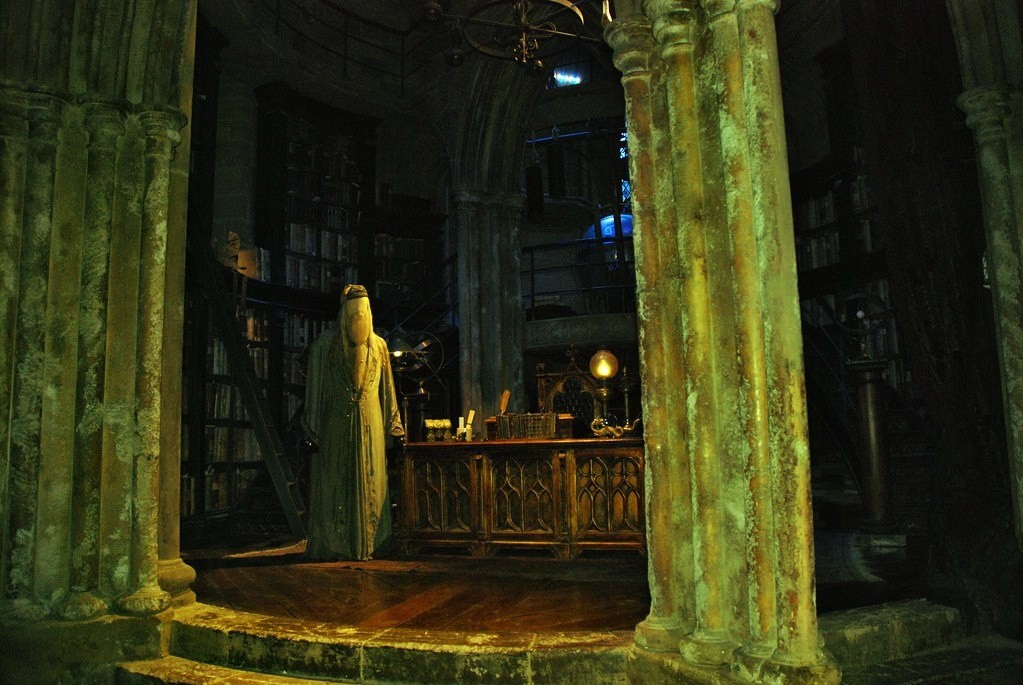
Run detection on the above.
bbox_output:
[534,343,598,432]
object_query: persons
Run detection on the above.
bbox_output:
[305,286,404,561]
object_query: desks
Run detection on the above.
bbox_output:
[395,436,648,557]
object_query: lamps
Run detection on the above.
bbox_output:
[589,349,619,425]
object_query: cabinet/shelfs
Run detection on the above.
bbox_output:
[180,11,459,544]
[790,146,913,396]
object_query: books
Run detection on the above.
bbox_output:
[181,118,425,521]
[494,411,558,440]
[795,142,916,410]
